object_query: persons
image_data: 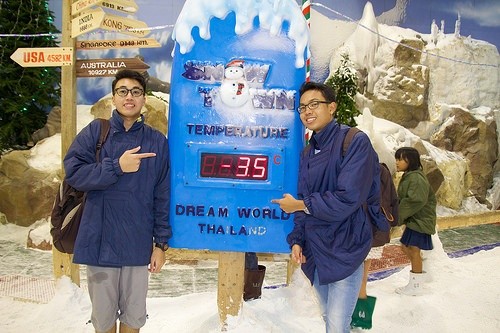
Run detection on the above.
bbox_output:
[395,147,437,296]
[270,81,389,333]
[244,252,266,301]
[350,245,386,329]
[62,70,172,333]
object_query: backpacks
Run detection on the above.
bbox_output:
[302,127,400,247]
[50,118,111,255]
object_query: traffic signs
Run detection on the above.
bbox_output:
[75,57,150,79]
[71,0,162,50]
[9,47,71,68]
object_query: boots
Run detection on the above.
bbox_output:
[350,295,376,329]
[395,269,427,297]
[243,265,266,302]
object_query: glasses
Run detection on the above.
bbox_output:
[297,101,333,114]
[114,87,145,97]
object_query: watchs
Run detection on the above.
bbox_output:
[304,207,310,215]
[155,242,169,251]
